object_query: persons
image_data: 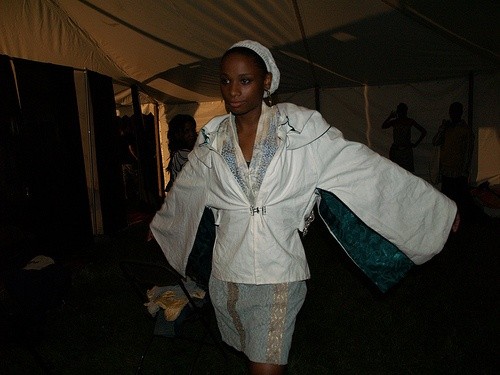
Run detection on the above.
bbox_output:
[153,112,210,335]
[380,103,428,175]
[433,102,473,203]
[146,40,460,375]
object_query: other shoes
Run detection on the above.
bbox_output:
[452,218,461,233]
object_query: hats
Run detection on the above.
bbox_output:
[219,40,280,98]
